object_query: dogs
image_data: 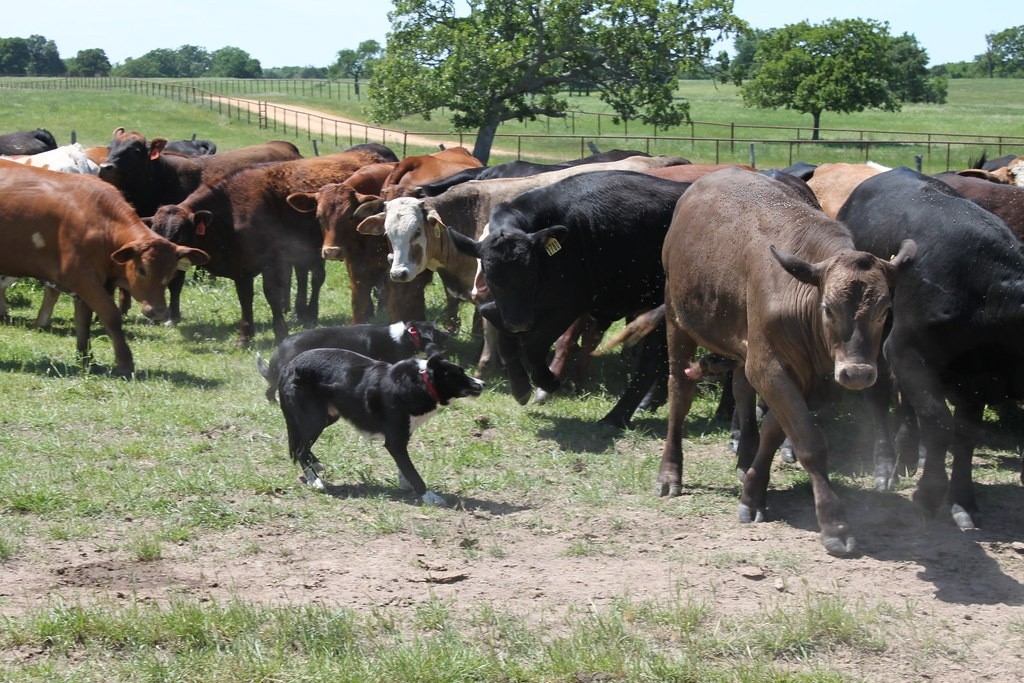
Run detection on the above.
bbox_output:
[256,319,453,406]
[278,348,486,508]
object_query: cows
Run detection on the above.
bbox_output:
[0,125,1020,563]
